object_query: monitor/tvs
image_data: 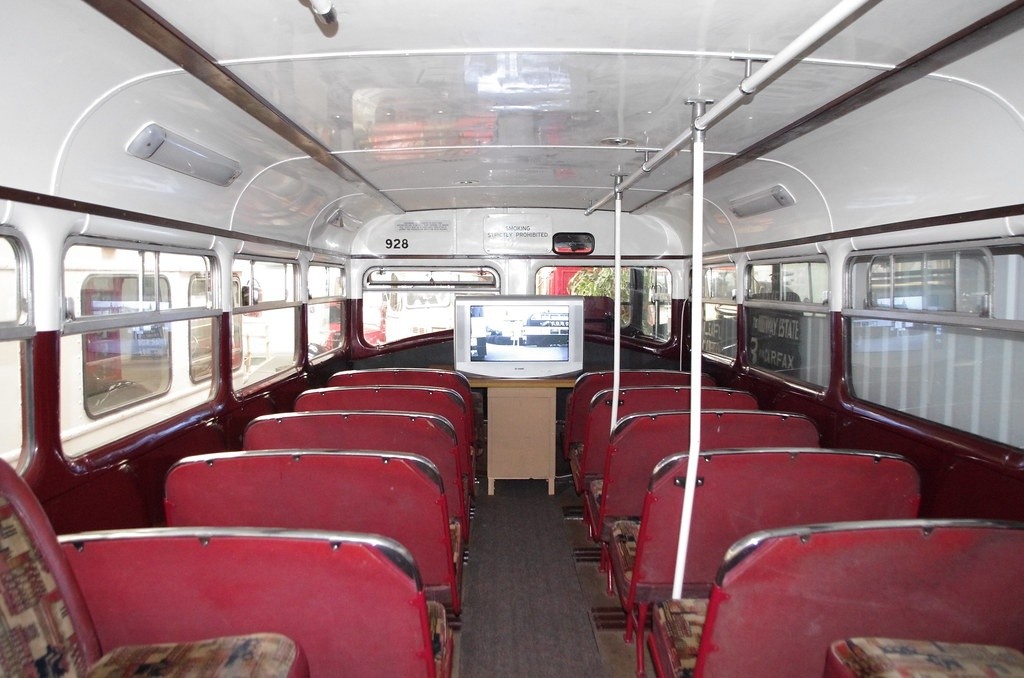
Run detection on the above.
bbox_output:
[454,295,584,379]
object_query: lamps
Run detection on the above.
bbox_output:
[728,184,796,218]
[125,122,243,188]
[326,210,363,232]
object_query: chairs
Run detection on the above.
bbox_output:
[564,369,1024,678]
[0,369,481,678]
